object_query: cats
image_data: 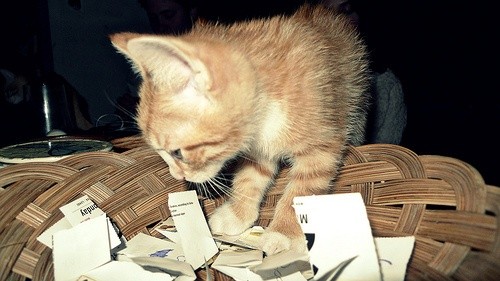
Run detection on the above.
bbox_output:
[109,3,371,257]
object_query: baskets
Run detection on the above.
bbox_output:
[0,133,500,281]
